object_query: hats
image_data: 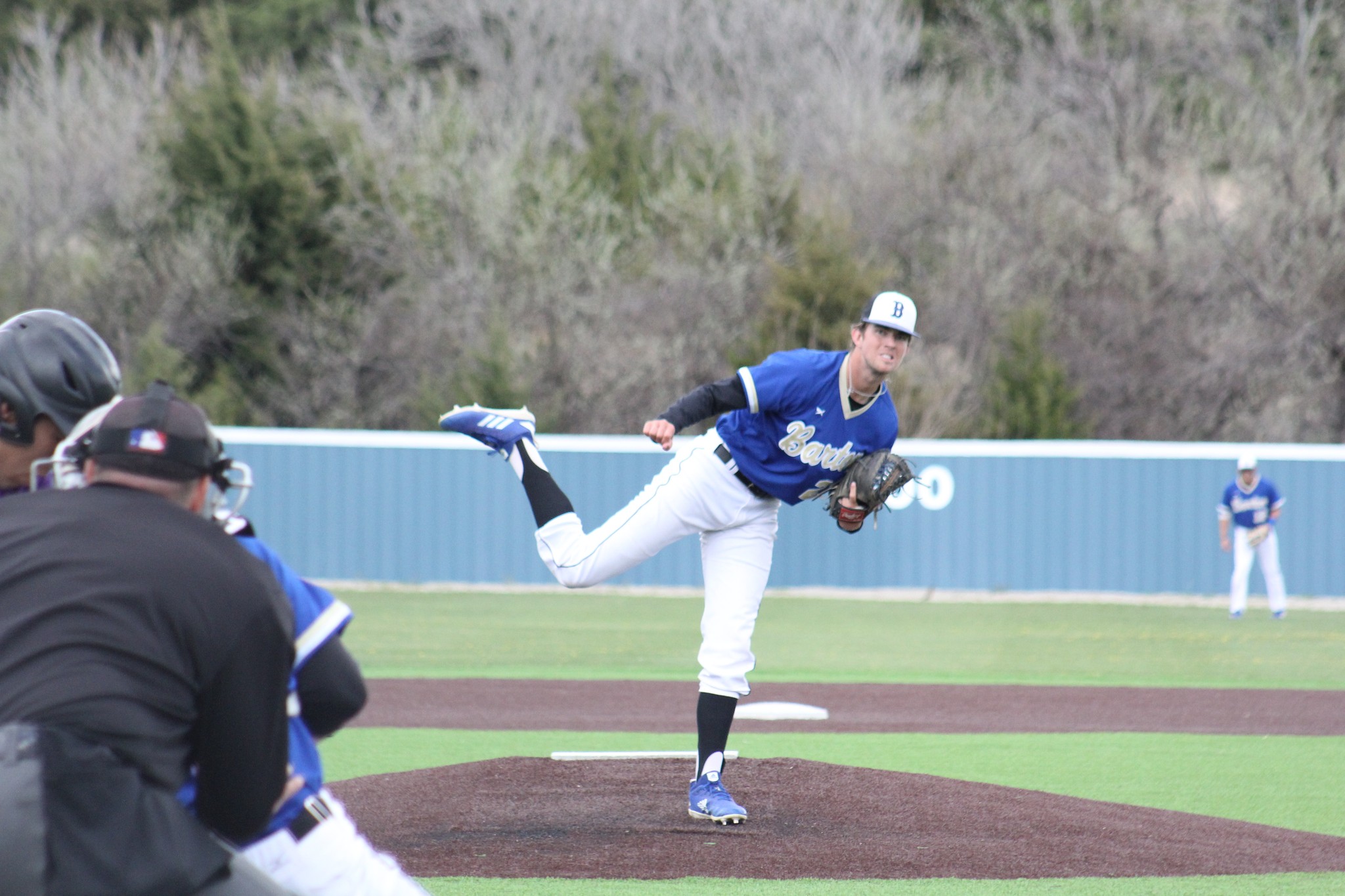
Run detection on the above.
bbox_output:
[1239,454,1256,470]
[85,390,233,494]
[863,290,918,339]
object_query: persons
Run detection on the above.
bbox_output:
[438,290,917,827]
[1217,453,1289,621]
[0,309,429,896]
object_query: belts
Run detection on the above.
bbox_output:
[715,445,775,500]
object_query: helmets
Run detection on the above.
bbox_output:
[0,308,121,445]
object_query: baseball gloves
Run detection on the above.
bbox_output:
[1251,523,1270,548]
[828,450,915,523]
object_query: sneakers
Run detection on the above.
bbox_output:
[688,770,747,825]
[437,402,538,460]
